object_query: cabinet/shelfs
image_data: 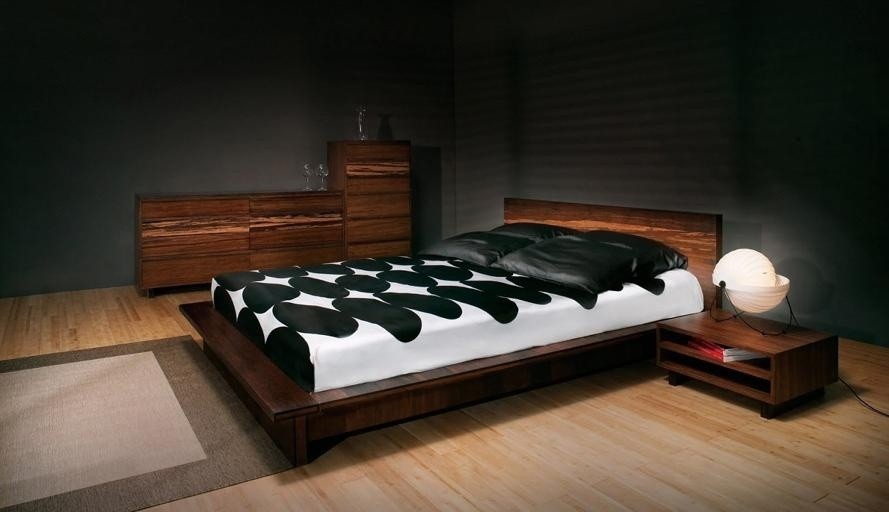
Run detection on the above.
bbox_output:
[134,190,346,299]
[326,138,412,259]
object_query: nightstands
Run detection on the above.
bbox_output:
[654,309,839,420]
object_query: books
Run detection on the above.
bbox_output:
[686,337,767,364]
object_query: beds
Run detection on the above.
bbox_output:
[178,197,724,467]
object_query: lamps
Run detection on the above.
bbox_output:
[709,248,792,336]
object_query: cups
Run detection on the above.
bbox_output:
[352,103,368,140]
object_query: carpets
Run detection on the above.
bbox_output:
[0,334,291,512]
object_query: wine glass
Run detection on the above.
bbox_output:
[315,161,329,191]
[303,160,313,192]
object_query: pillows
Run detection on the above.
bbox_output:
[580,230,691,278]
[489,237,636,295]
[485,222,583,241]
[416,232,535,267]
